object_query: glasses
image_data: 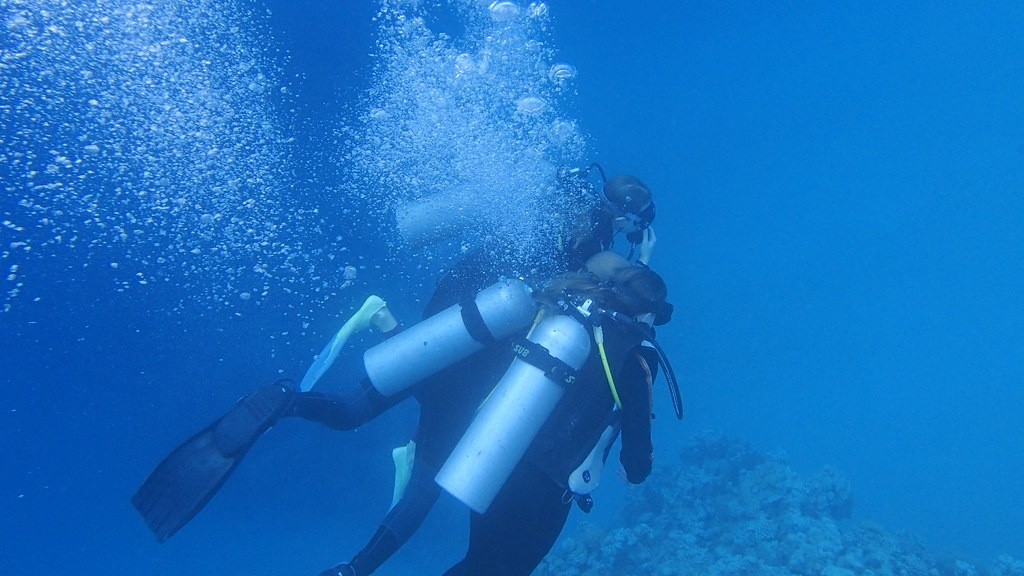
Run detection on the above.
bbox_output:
[652,301,674,326]
[633,201,656,230]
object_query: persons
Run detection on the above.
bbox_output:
[132,173,674,576]
